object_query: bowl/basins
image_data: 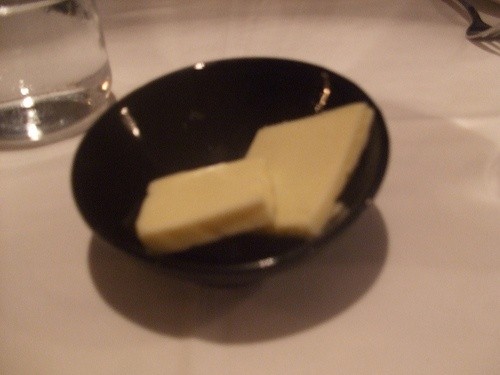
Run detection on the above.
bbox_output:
[70,58,389,287]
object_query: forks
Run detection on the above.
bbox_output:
[453,0,500,40]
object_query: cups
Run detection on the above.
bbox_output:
[0,0,112,145]
